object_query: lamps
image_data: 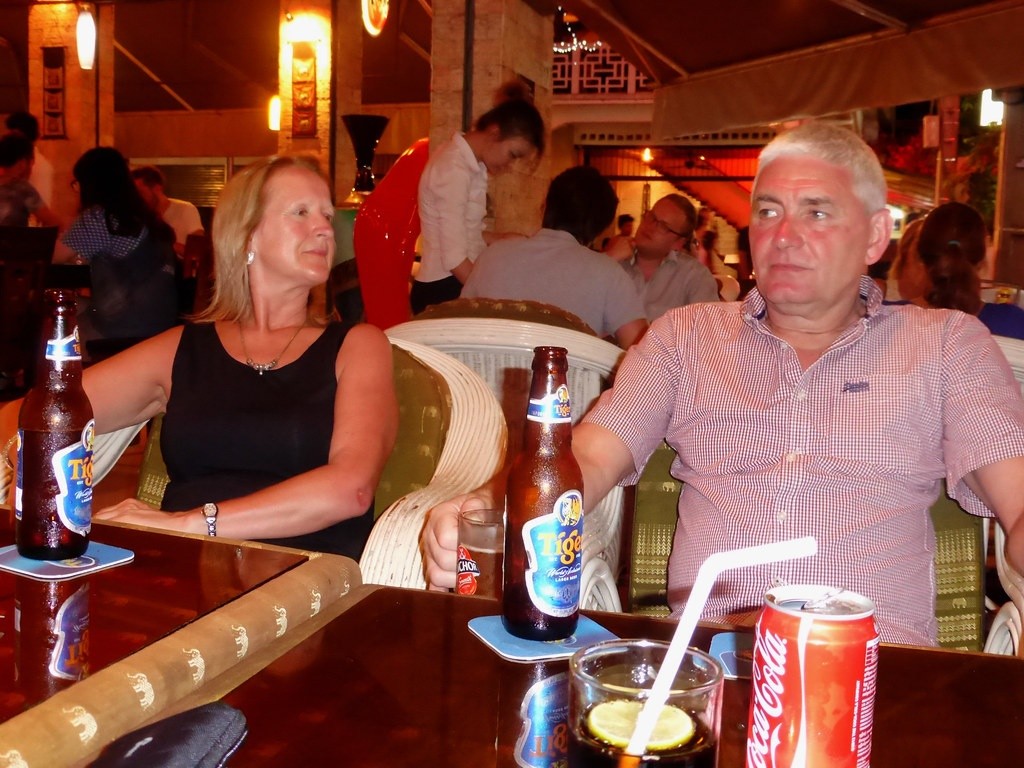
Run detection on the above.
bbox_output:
[267,94,285,131]
[76,2,96,70]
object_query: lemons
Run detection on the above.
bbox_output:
[586,700,695,751]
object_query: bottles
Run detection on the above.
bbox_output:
[502,346,585,640]
[16,289,96,560]
[14,576,89,704]
[496,661,566,768]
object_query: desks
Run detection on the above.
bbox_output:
[0,502,1024,768]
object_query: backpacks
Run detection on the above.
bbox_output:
[90,227,187,341]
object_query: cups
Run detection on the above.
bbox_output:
[456,509,507,600]
[565,638,723,768]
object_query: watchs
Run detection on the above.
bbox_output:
[200,502,219,536]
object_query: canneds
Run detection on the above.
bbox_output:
[743,584,880,768]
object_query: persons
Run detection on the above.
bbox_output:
[418,122,1024,647]
[409,79,546,315]
[0,154,399,562]
[0,110,204,445]
[457,165,1024,349]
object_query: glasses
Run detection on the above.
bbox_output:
[252,154,324,217]
[71,181,79,191]
[641,209,688,239]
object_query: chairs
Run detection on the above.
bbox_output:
[85,233,1024,655]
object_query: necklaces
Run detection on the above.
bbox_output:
[239,318,304,375]
